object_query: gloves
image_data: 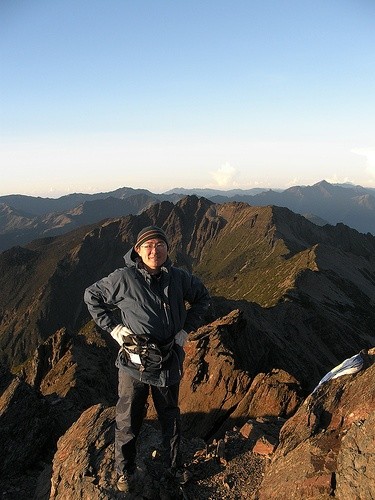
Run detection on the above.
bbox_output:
[174,329,188,347]
[111,324,133,347]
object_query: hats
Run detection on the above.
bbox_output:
[134,226,168,248]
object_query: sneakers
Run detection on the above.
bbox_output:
[115,476,128,491]
[168,470,190,484]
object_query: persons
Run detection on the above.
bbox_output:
[83,225,212,490]
